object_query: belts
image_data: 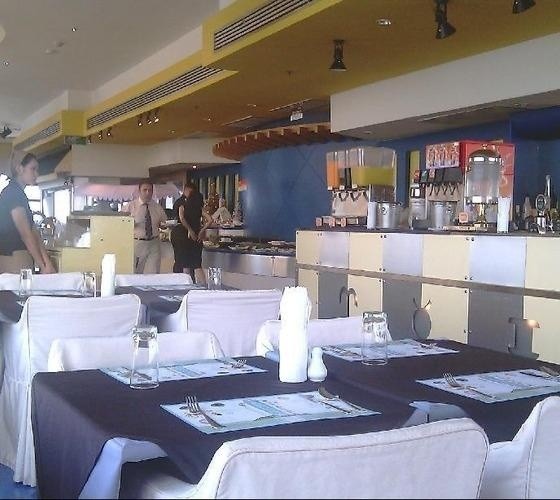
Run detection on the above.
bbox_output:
[134,236,158,240]
[178,222,181,224]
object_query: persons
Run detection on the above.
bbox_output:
[178,190,213,287]
[0,147,58,278]
[116,180,169,274]
[171,179,191,275]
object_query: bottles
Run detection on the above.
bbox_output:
[522,195,531,220]
[307,346,327,382]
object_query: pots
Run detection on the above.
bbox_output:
[367,201,401,230]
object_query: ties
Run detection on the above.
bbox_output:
[145,203,152,238]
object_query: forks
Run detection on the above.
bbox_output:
[442,371,498,400]
[213,358,247,369]
[184,394,224,432]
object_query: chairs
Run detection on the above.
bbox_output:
[0,272,560,500]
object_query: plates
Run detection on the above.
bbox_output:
[228,240,296,255]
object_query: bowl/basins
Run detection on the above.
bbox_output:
[221,238,231,242]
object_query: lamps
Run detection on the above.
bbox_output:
[434,0,456,39]
[328,41,346,71]
[513,0,536,14]
[87,109,160,144]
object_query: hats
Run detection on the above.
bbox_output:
[7,151,30,179]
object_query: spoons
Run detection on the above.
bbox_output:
[317,386,362,412]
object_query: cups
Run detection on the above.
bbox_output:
[128,324,161,390]
[361,311,390,367]
[81,272,97,299]
[166,220,178,225]
[18,269,33,299]
[208,267,222,290]
